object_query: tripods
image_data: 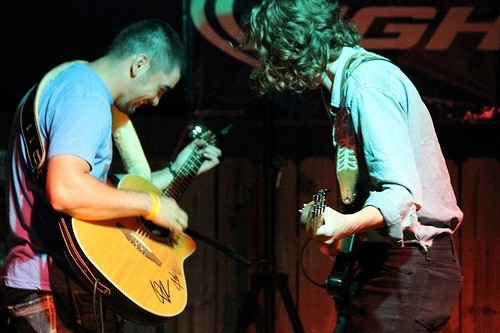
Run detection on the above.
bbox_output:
[236,108,306,333]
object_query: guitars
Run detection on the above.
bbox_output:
[298,189,364,319]
[52,125,218,325]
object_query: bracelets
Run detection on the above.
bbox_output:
[166,160,177,178]
[143,192,160,221]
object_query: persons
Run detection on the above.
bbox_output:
[4,18,222,333]
[227,0,465,333]
